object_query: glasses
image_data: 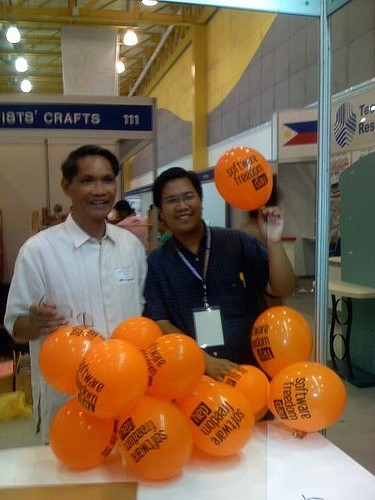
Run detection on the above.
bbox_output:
[159,192,199,206]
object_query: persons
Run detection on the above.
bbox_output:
[115,205,146,247]
[147,204,154,251]
[237,186,283,307]
[4,144,148,445]
[143,167,307,439]
[46,203,67,226]
[157,212,174,243]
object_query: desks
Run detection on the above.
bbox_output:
[1,419,374,500]
[327,281,375,388]
[302,236,317,277]
[327,256,342,312]
[282,237,296,267]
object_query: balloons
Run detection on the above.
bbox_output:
[51,398,117,469]
[40,325,104,395]
[116,394,193,479]
[186,381,255,455]
[215,146,273,211]
[268,362,346,431]
[224,365,269,413]
[145,333,205,404]
[76,339,147,418]
[112,316,162,353]
[252,305,313,378]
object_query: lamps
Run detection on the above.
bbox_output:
[115,0,158,74]
